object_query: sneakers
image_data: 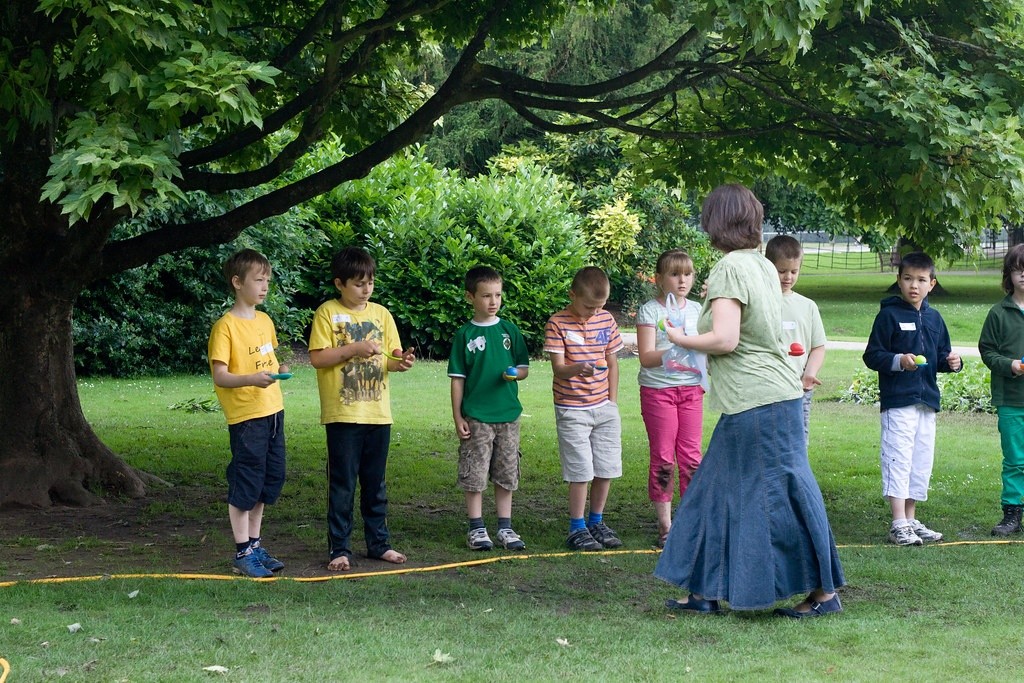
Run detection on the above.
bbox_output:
[232,548,274,578]
[467,527,494,551]
[589,521,623,549]
[493,528,525,551]
[886,522,923,546]
[908,520,943,542]
[252,546,285,572]
[565,527,603,551]
[657,532,669,549]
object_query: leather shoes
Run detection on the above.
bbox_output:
[665,593,721,615]
[774,592,844,619]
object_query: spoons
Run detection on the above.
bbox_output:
[270,372,292,381]
[1020,363,1024,370]
[666,360,701,375]
[591,365,609,370]
[506,375,515,380]
[788,350,805,356]
[658,320,668,333]
[915,363,929,367]
[382,350,404,360]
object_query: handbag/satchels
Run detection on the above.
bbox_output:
[657,292,703,385]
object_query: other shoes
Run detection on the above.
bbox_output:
[991,504,1024,537]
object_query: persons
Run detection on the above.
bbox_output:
[308,248,415,572]
[545,265,624,550]
[862,253,963,547]
[207,249,289,578]
[636,251,709,548]
[446,265,529,550]
[978,243,1024,536]
[765,236,828,454]
[652,183,846,618]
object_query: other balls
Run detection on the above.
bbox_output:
[278,365,290,374]
[506,366,519,376]
[790,343,804,351]
[914,354,927,364]
[596,359,607,366]
[1021,356,1024,364]
[658,313,682,332]
[392,349,402,358]
